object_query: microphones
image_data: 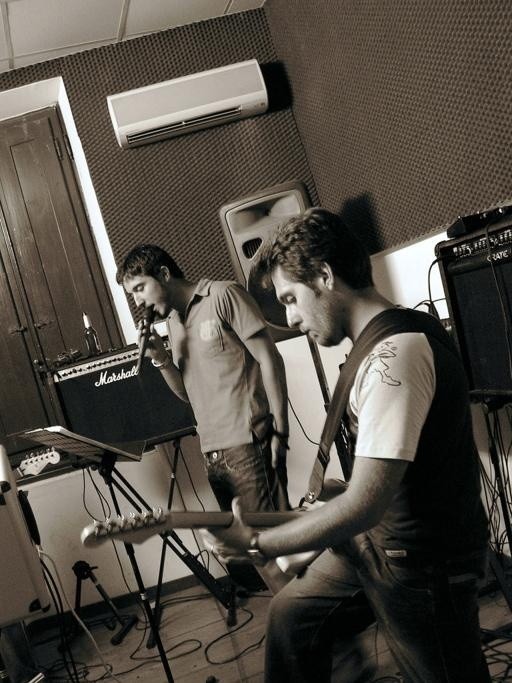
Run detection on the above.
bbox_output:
[135,307,156,373]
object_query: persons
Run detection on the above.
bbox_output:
[114,245,288,510]
[200,207,495,683]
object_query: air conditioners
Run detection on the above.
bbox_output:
[106,58,269,150]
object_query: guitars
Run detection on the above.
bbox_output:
[81,480,350,575]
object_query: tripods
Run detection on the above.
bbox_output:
[95,465,235,651]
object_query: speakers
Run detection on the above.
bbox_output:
[434,205,512,398]
[219,180,314,345]
[50,336,199,447]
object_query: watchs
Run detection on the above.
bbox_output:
[247,527,269,569]
[151,353,172,368]
